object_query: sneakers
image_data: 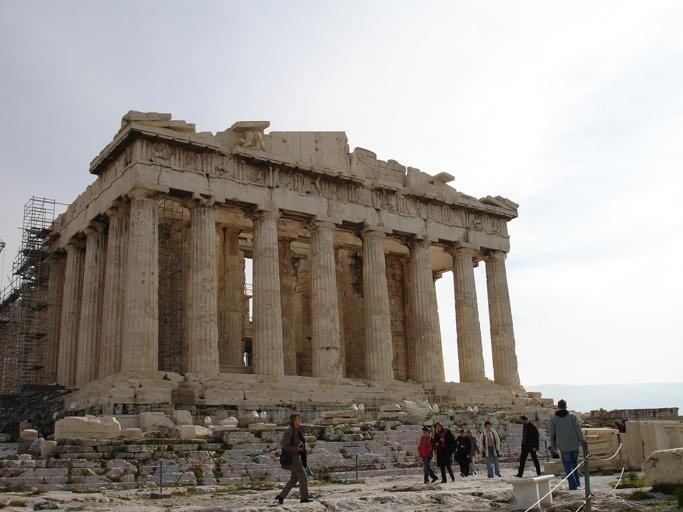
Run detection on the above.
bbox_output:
[441,481,446,483]
[459,473,477,477]
[514,474,522,477]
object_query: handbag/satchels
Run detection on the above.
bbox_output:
[279,449,292,469]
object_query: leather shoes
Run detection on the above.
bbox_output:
[275,495,283,504]
[431,478,438,483]
[300,499,313,503]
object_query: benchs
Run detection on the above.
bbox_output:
[506,473,556,510]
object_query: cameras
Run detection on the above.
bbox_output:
[299,442,304,448]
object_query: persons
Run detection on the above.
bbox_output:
[416,426,441,485]
[548,399,588,491]
[272,412,315,504]
[479,421,502,479]
[455,428,471,478]
[591,416,626,432]
[513,414,542,477]
[466,427,480,477]
[434,421,457,483]
